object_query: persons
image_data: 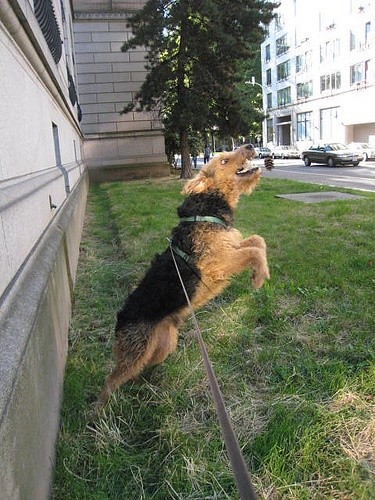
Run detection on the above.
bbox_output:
[203,144,211,165]
[193,153,198,169]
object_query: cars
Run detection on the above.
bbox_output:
[253,147,274,158]
[217,146,232,151]
[300,141,366,167]
[346,141,375,163]
[272,145,302,158]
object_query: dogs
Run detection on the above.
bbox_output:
[94,143,270,417]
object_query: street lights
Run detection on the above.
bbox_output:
[245,77,263,93]
